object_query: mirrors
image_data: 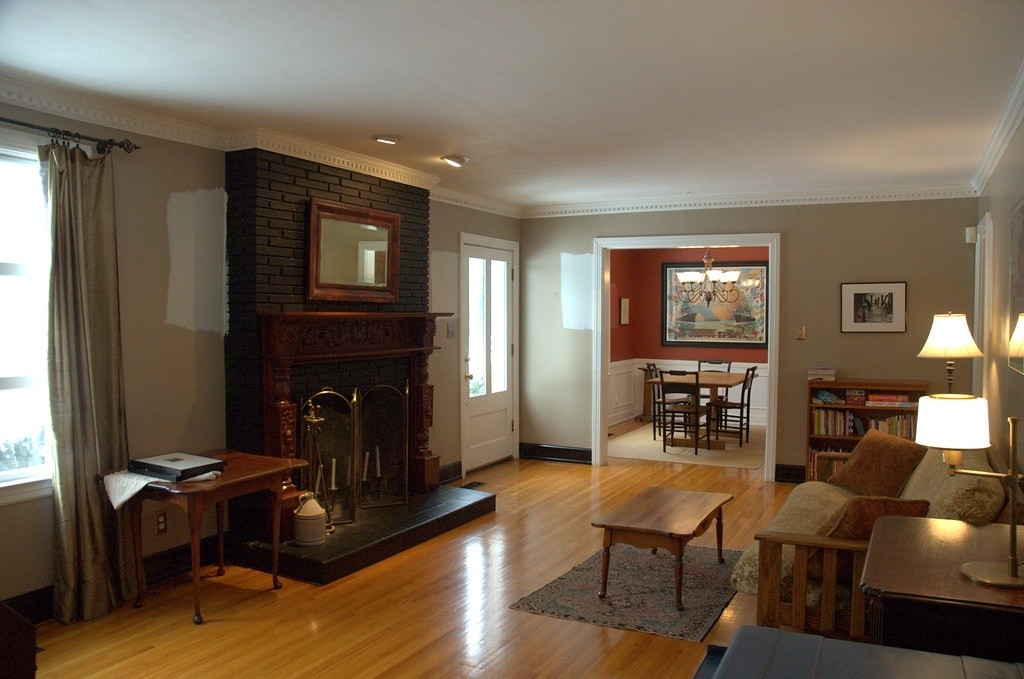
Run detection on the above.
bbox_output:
[1007,197,1024,375]
[308,196,401,304]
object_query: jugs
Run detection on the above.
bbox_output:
[293,492,327,547]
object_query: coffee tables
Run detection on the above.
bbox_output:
[591,486,734,613]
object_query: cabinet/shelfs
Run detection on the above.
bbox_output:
[804,376,930,481]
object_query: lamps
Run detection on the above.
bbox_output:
[376,135,398,145]
[916,309,986,394]
[441,155,469,167]
[915,394,1024,590]
[1009,313,1024,361]
[678,249,739,307]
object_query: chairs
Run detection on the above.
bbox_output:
[657,369,711,455]
[646,362,691,440]
[706,365,758,450]
[687,360,733,431]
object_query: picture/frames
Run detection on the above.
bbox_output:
[661,260,769,348]
[840,281,907,333]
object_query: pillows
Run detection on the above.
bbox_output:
[826,429,929,498]
[789,497,930,578]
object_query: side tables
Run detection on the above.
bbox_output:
[860,513,1024,662]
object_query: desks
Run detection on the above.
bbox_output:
[96,448,310,625]
[647,372,759,450]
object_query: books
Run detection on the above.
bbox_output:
[832,461,843,474]
[868,414,914,438]
[865,394,918,407]
[808,447,817,480]
[807,367,836,381]
[811,407,853,435]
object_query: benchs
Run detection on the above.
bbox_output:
[731,448,1024,643]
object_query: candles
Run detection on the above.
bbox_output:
[314,446,382,494]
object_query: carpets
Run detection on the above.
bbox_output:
[508,545,745,645]
